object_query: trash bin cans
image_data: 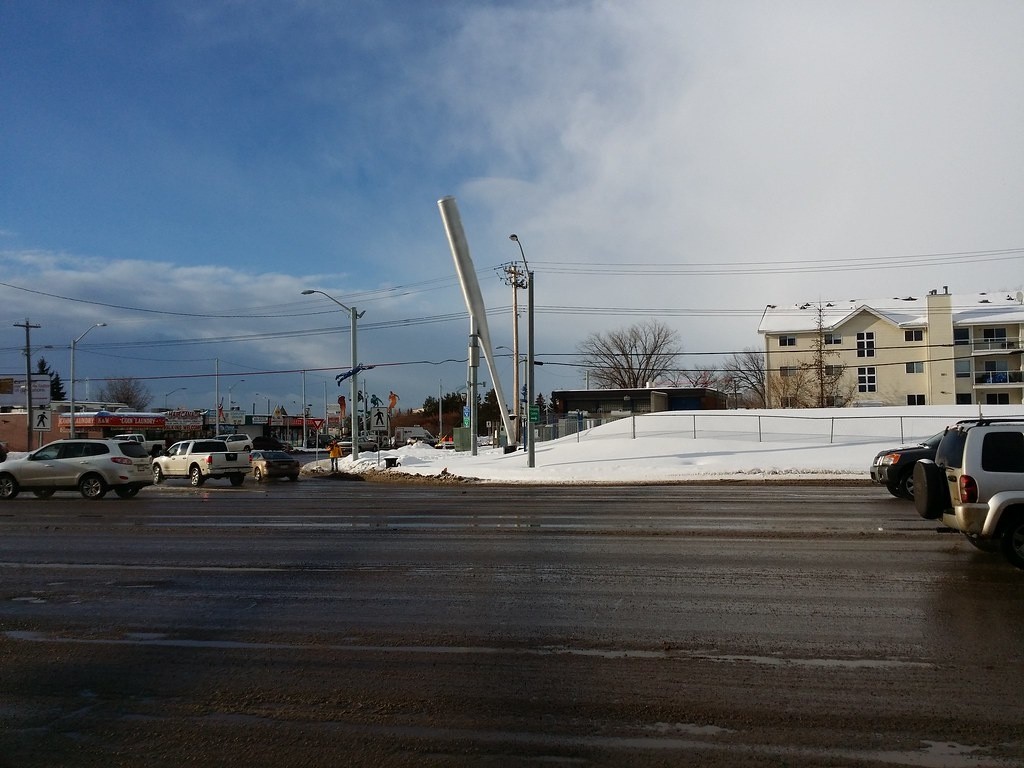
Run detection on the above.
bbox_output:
[383,458,398,469]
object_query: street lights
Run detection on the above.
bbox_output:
[228,380,246,410]
[256,393,271,413]
[26,344,54,453]
[508,233,536,469]
[165,387,187,408]
[301,289,365,461]
[68,321,107,438]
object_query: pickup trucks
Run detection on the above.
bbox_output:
[151,438,252,487]
[337,438,378,454]
[113,434,166,453]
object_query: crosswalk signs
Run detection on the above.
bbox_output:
[32,410,52,432]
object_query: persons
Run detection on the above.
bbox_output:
[327,438,343,474]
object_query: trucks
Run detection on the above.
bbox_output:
[394,425,435,446]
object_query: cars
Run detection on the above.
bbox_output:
[250,450,300,482]
[307,434,336,448]
[212,435,254,452]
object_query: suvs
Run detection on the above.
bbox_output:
[869,426,947,501]
[253,435,293,450]
[912,418,1024,568]
[0,438,154,499]
